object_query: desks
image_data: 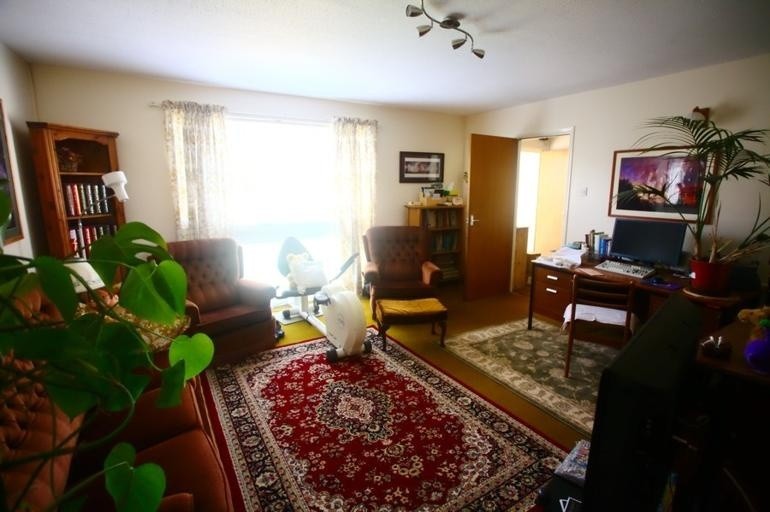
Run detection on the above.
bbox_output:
[528,247,768,365]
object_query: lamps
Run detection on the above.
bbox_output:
[77,170,129,247]
[406,0,485,59]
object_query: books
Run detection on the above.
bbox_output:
[579,266,604,277]
[554,437,591,488]
[584,231,612,256]
[424,209,461,280]
[62,183,118,260]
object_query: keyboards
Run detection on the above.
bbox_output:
[594,259,656,279]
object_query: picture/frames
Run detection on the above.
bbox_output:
[608,144,720,225]
[399,149,445,183]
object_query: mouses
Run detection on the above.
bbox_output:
[652,277,664,283]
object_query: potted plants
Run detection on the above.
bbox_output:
[610,114,770,299]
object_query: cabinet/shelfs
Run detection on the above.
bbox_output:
[532,264,581,331]
[405,204,466,287]
[26,119,130,304]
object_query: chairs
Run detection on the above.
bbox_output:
[147,237,277,367]
[360,225,443,320]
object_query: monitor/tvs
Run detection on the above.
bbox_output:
[609,217,688,266]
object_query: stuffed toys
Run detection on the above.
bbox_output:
[735,303,769,368]
[277,251,329,296]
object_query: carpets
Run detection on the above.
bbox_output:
[195,324,571,512]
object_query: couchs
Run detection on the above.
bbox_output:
[0,274,234,512]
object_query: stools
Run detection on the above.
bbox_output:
[375,298,448,351]
